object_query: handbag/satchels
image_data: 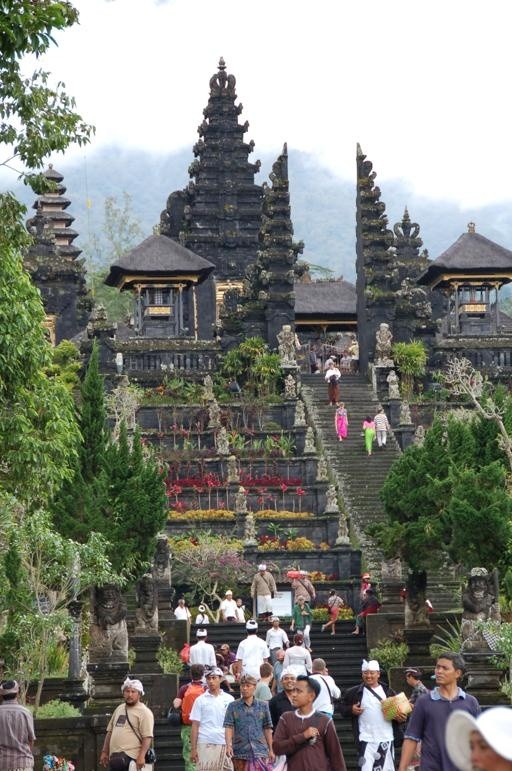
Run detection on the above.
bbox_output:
[146,748,156,764]
[107,751,131,771]
[270,590,275,600]
[327,607,331,615]
[392,722,405,748]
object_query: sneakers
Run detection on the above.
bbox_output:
[307,647,313,653]
[320,625,360,636]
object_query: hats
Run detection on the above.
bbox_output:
[220,644,230,650]
[444,707,512,771]
[362,573,370,580]
[275,649,286,661]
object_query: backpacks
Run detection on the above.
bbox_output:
[182,684,205,727]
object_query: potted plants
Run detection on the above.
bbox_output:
[390,334,431,400]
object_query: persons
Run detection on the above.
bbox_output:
[373,406,390,448]
[361,416,376,456]
[0,679,36,771]
[324,362,341,406]
[334,402,349,442]
[98,563,512,771]
[309,334,359,376]
